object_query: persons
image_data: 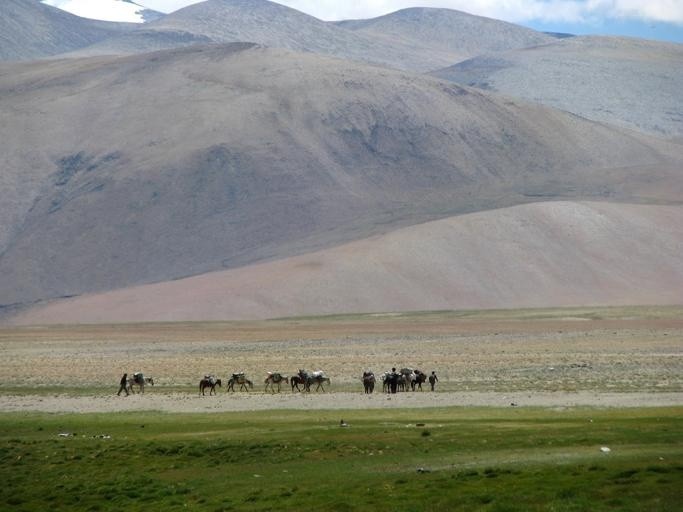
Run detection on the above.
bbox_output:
[429,371,439,391]
[390,367,398,393]
[117,373,130,396]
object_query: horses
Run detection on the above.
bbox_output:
[290,376,331,392]
[226,377,254,393]
[262,375,289,393]
[199,378,222,396]
[380,370,427,392]
[126,376,154,393]
[361,375,375,395]
